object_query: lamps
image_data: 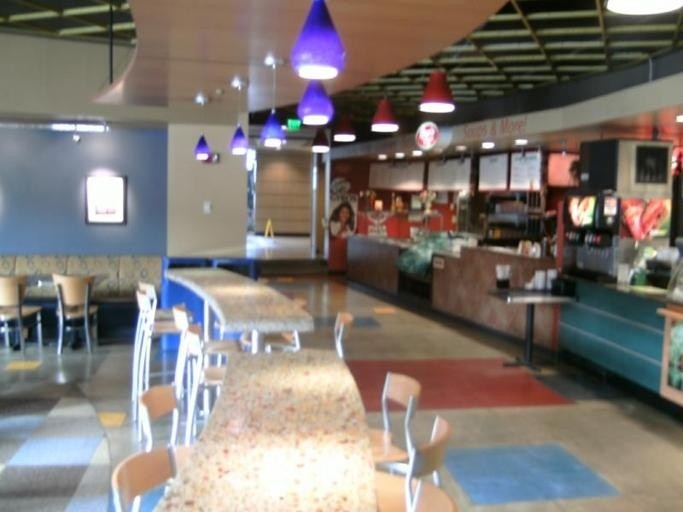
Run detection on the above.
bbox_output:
[369,100,400,134]
[296,82,335,127]
[227,74,252,158]
[191,91,213,162]
[289,1,349,81]
[258,50,287,151]
[332,112,357,143]
[416,68,456,116]
[311,128,331,155]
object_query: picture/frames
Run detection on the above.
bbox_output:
[83,169,130,228]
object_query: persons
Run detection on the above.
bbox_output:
[329,199,354,240]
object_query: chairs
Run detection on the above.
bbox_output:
[0,272,43,357]
[50,271,99,357]
[109,267,455,512]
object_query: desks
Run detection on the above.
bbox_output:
[485,287,579,375]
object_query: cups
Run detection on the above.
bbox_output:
[616,263,630,283]
[493,263,511,288]
[534,267,557,291]
